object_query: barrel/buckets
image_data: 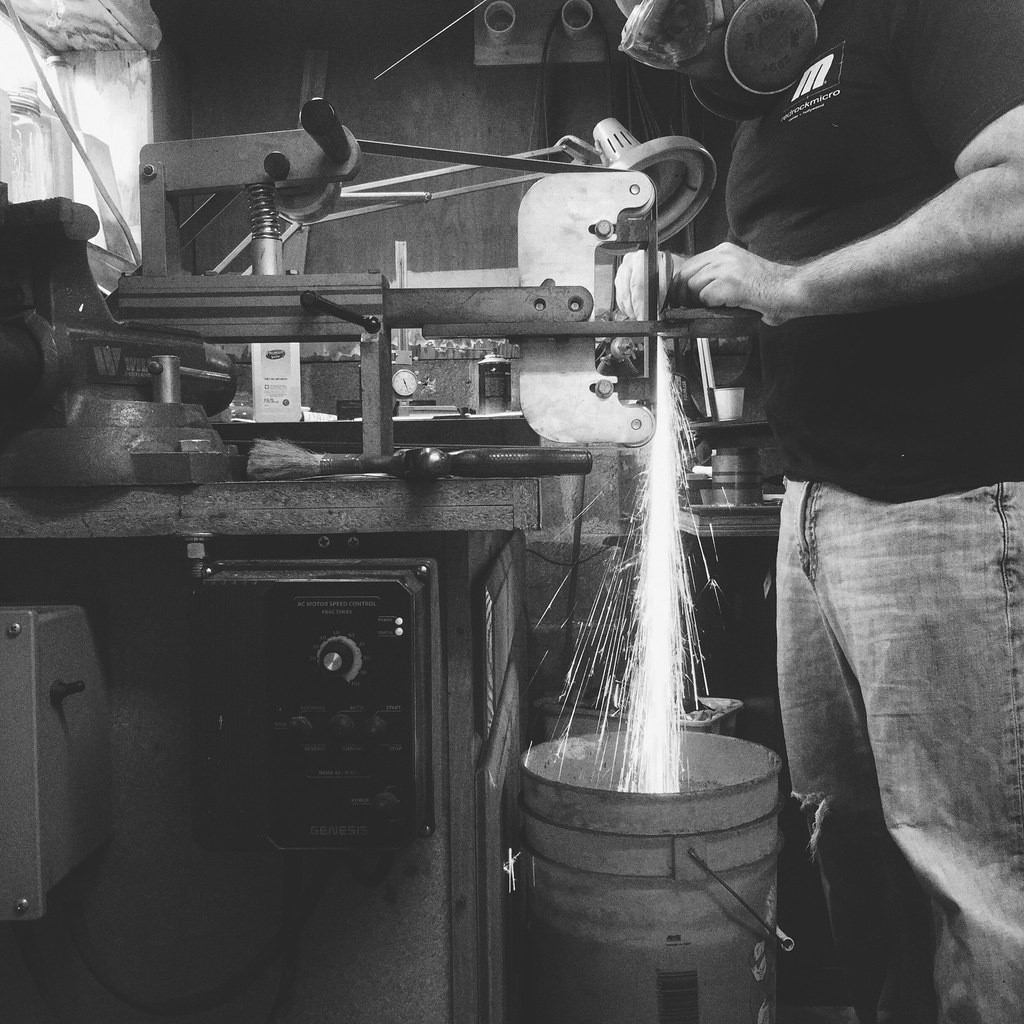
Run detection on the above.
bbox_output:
[519,730,794,1024]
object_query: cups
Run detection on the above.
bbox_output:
[713,388,744,421]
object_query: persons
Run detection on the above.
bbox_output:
[616,0,1024,1023]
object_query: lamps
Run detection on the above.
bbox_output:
[214,116,718,277]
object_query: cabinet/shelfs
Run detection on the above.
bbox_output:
[0,463,585,1024]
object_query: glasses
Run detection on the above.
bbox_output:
[618,0,715,72]
